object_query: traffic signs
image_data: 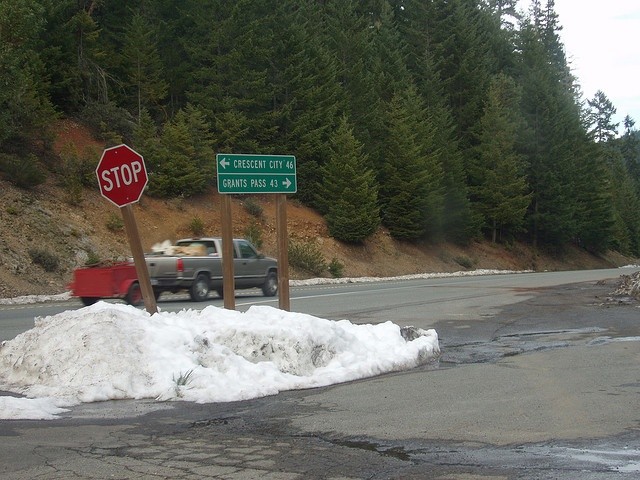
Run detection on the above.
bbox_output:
[216,153,298,193]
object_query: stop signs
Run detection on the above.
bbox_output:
[94,144,149,208]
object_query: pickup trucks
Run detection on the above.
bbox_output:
[129,237,283,300]
